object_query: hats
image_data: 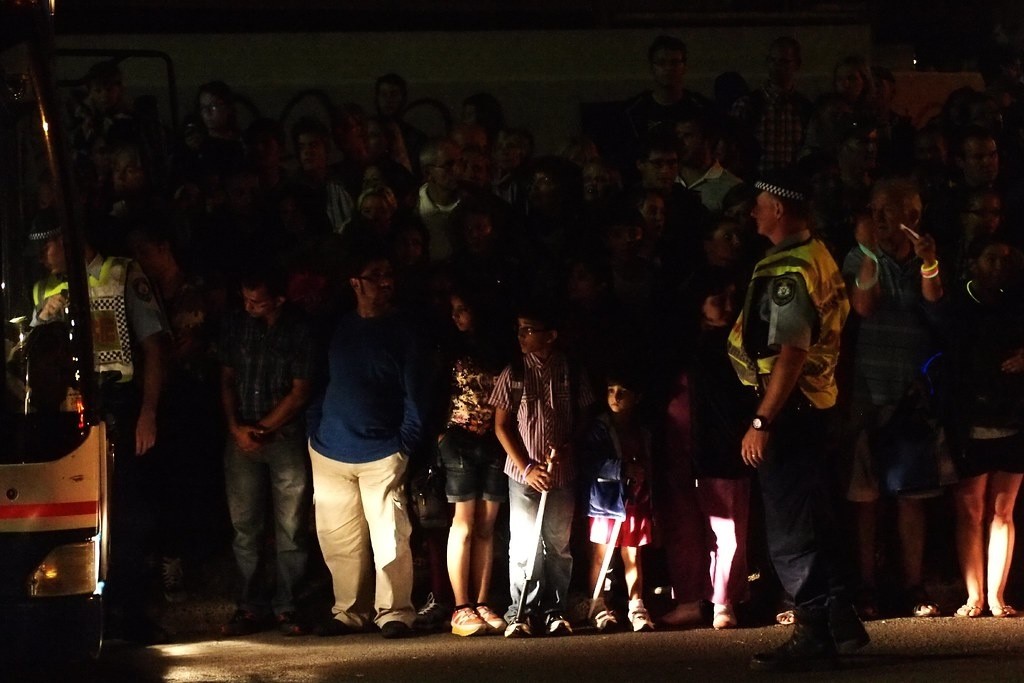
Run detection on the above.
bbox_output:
[23,211,64,246]
[744,164,817,206]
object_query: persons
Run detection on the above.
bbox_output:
[1,34,1024,636]
[726,171,871,666]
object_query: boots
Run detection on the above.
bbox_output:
[826,590,872,655]
[750,605,841,673]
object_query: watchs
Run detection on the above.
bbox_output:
[750,416,773,433]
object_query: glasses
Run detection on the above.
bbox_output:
[356,272,395,287]
[200,103,225,115]
[517,323,552,336]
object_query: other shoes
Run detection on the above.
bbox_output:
[473,603,507,634]
[591,610,618,633]
[451,606,488,637]
[313,618,368,637]
[503,614,533,639]
[542,610,574,635]
[272,610,307,636]
[382,621,411,639]
[626,607,655,631]
[854,591,1016,619]
[410,591,456,631]
[660,600,796,629]
[217,609,258,636]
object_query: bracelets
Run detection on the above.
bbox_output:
[920,261,940,279]
[522,463,532,480]
